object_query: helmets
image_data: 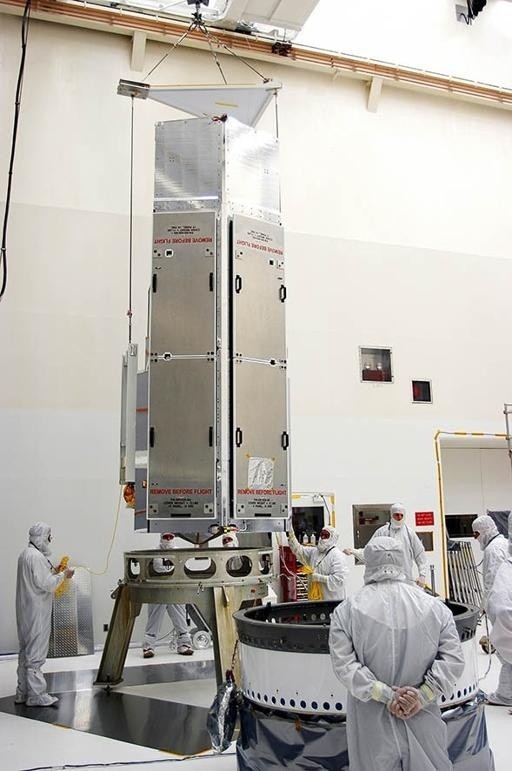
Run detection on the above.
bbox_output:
[472,516,499,550]
[29,522,53,555]
[363,536,409,583]
[222,532,236,548]
[318,525,339,553]
[159,532,175,550]
[390,503,407,530]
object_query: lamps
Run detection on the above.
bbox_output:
[362,361,384,380]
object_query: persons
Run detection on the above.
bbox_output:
[143,532,194,658]
[482,513,511,705]
[287,524,346,600]
[472,515,508,611]
[343,503,428,588]
[222,532,239,546]
[15,522,74,706]
[328,537,465,770]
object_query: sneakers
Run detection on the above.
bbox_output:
[15,692,28,703]
[26,695,59,706]
[177,645,194,654]
[143,649,154,657]
[487,693,512,706]
